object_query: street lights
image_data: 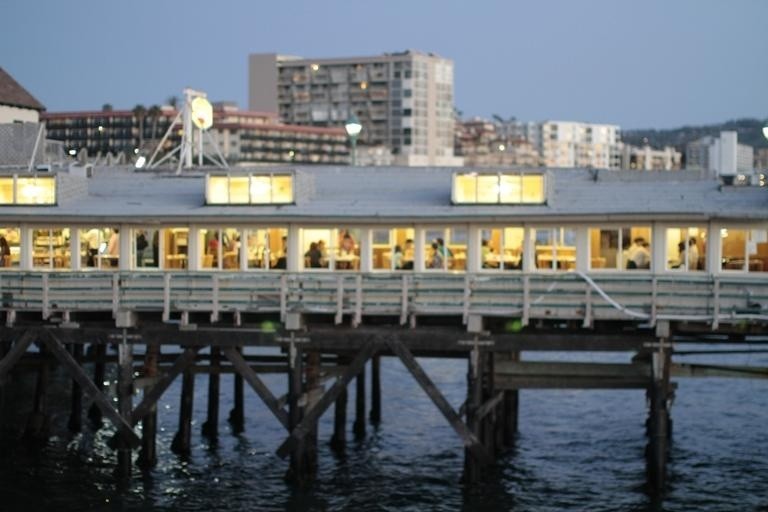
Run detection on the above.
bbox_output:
[343,111,364,168]
[759,120,767,140]
[68,147,79,157]
[495,140,506,164]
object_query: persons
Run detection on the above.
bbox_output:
[339,234,354,255]
[481,240,494,267]
[674,238,700,270]
[62,227,159,267]
[274,236,287,269]
[1,235,10,267]
[211,231,227,268]
[305,240,326,267]
[391,239,414,269]
[430,238,451,269]
[626,237,651,269]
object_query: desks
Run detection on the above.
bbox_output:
[0,227,767,271]
